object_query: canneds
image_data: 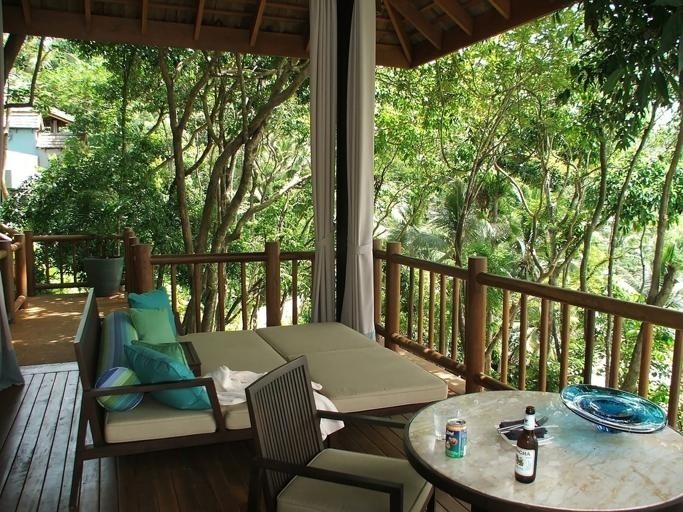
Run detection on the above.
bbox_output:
[446,418,467,458]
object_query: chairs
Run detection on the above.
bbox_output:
[244,354,435,512]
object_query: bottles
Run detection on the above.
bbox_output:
[513,405,537,484]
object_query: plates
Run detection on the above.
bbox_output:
[559,384,668,434]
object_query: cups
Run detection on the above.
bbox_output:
[433,407,462,442]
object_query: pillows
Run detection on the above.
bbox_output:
[95,311,144,412]
[128,307,177,344]
[131,340,189,369]
[127,286,178,336]
[123,344,213,411]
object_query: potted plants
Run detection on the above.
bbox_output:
[65,201,133,297]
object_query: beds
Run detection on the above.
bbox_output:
[69,286,449,511]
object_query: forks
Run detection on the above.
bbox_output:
[496,415,550,435]
